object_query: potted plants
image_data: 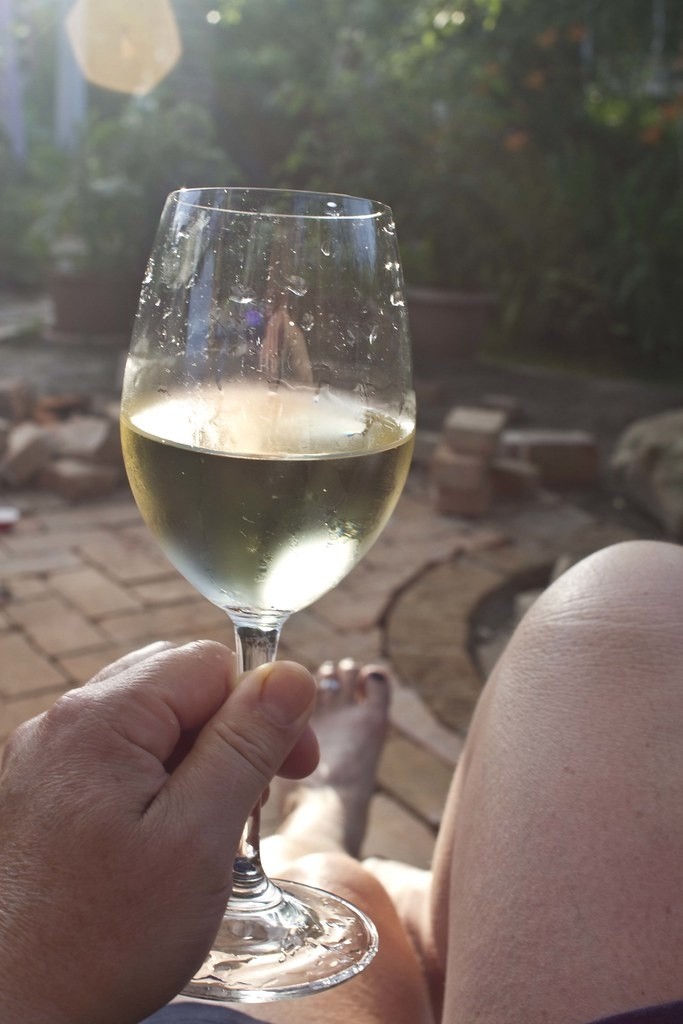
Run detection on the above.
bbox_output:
[47,275,479,359]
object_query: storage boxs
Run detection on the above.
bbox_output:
[2,384,137,500]
[430,407,601,515]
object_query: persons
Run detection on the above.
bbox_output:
[0,540,683,1024]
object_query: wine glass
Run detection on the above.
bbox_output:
[121,184,415,1002]
